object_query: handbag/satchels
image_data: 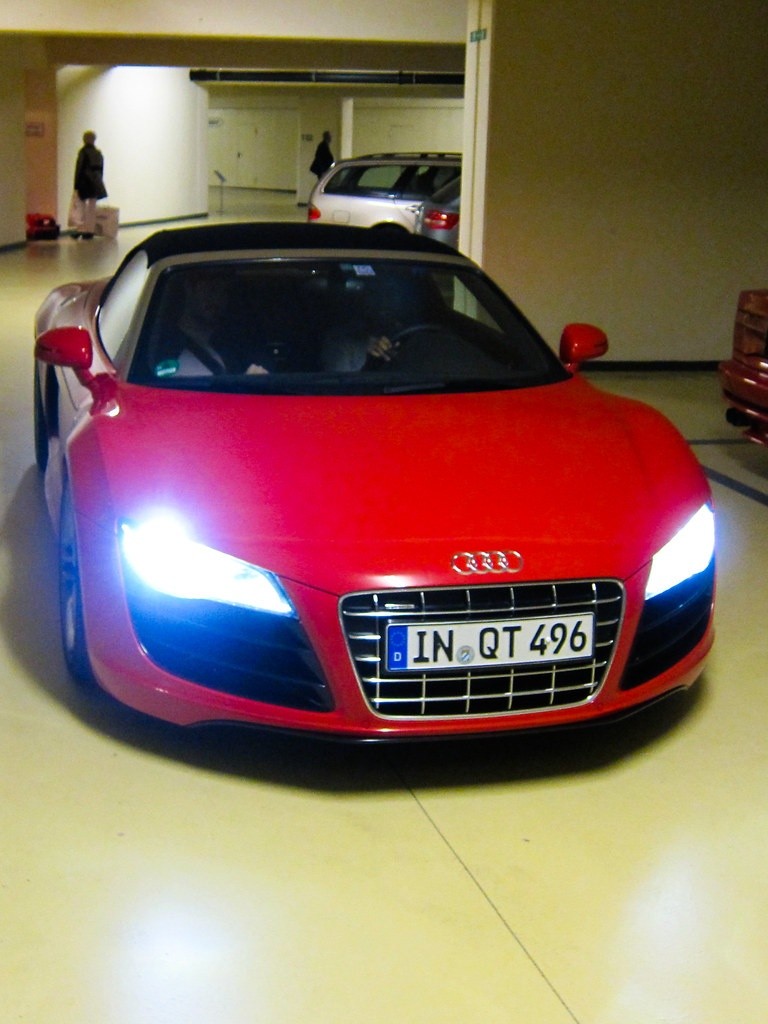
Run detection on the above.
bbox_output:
[94,198,119,238]
[68,191,85,227]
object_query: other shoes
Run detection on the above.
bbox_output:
[71,232,80,239]
[82,233,93,239]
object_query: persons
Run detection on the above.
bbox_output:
[311,131,334,180]
[70,131,107,240]
[320,260,450,371]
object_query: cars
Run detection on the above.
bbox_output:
[720,289,767,449]
[33,223,719,781]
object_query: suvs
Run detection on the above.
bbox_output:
[309,151,461,250]
